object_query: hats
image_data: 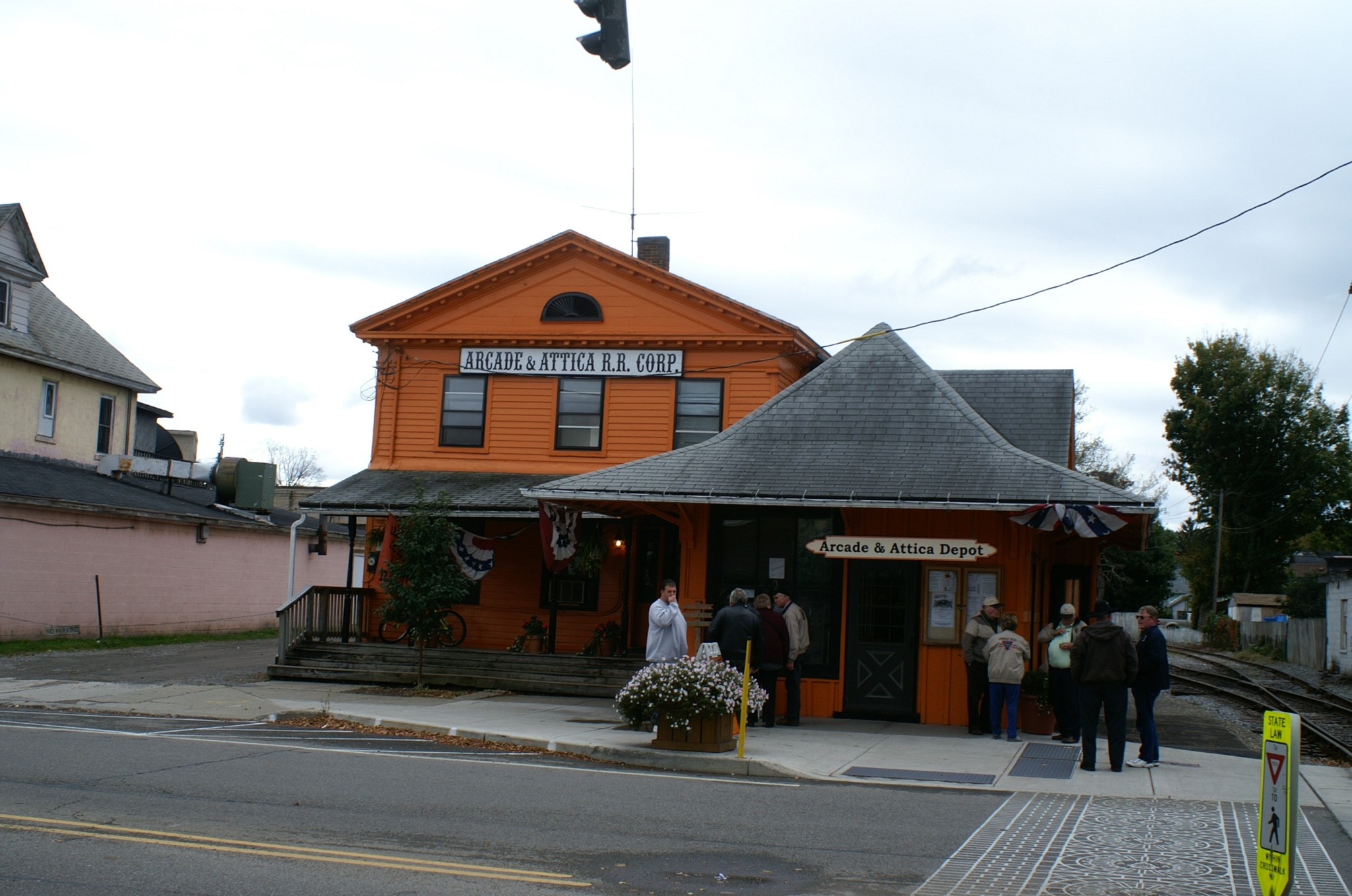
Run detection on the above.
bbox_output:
[982,596,1004,606]
[774,581,793,599]
[1061,604,1075,614]
[1088,600,1114,617]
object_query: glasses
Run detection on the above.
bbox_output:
[1136,615,1152,620]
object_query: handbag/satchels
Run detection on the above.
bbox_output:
[1022,670,1041,695]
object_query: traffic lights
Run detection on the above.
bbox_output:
[574,0,630,70]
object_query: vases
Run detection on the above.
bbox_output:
[1019,692,1056,735]
[527,635,543,653]
[652,706,737,753]
[599,638,613,657]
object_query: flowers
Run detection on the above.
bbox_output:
[1022,669,1051,716]
[612,656,769,732]
[575,618,626,657]
[506,614,548,653]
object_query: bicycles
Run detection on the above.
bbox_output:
[377,578,466,648]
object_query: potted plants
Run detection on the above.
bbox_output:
[410,627,438,648]
[366,526,384,551]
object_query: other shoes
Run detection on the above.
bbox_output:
[993,734,1001,739]
[1063,736,1080,742]
[1052,734,1065,739]
[1007,737,1024,741]
[969,729,984,735]
[747,722,754,727]
[1112,766,1122,772]
[762,722,774,727]
[653,726,658,732]
[1081,763,1095,771]
[776,718,800,726]
[982,728,993,733]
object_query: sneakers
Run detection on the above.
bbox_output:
[1125,758,1155,768]
[1155,761,1159,767]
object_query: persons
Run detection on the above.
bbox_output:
[1069,599,1136,772]
[1036,603,1089,743]
[705,587,761,728]
[1124,605,1170,768]
[646,580,688,668]
[774,590,812,728]
[983,614,1030,742]
[744,593,789,727]
[962,596,1004,735]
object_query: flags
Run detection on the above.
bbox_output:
[538,499,582,571]
[445,523,534,580]
[1001,503,1130,538]
